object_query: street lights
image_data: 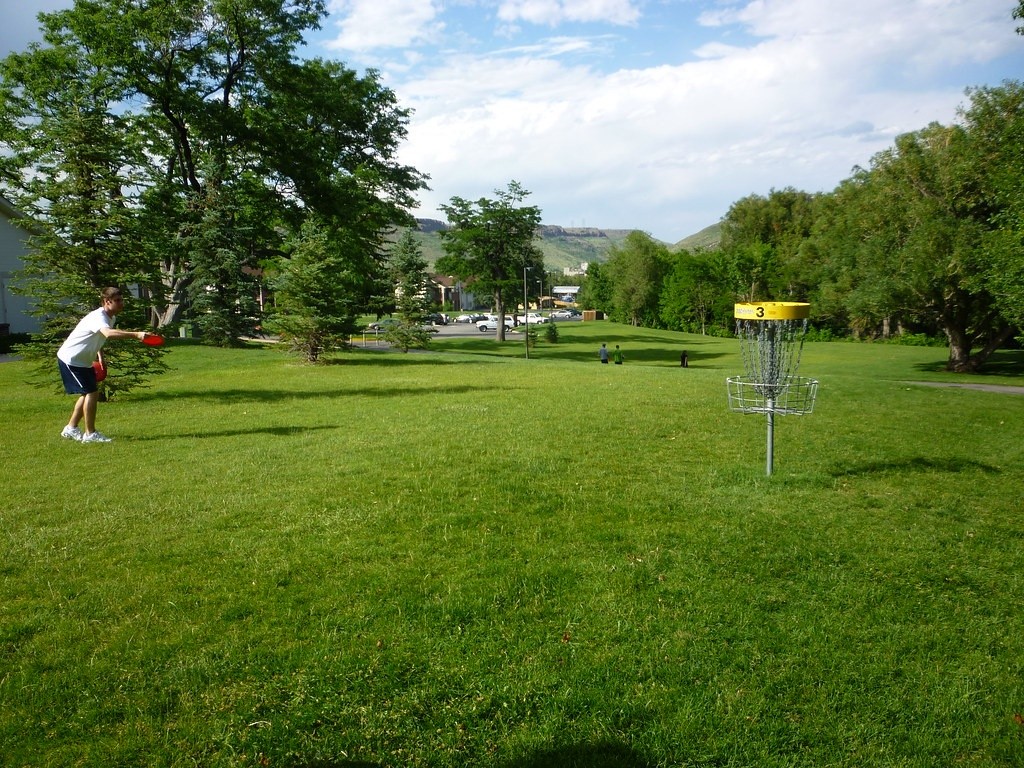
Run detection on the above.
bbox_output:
[536,279,542,317]
[524,266,535,358]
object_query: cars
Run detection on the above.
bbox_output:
[418,312,492,328]
[370,318,407,331]
[548,309,573,319]
[567,308,582,317]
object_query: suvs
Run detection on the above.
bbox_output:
[476,316,515,333]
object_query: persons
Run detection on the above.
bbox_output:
[680,350,688,367]
[598,343,610,364]
[57,287,152,443]
[612,345,625,365]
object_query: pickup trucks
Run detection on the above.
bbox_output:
[516,313,549,327]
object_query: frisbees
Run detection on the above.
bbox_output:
[92,361,107,382]
[142,334,163,345]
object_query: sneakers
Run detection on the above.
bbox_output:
[81,430,112,442]
[60,424,83,441]
